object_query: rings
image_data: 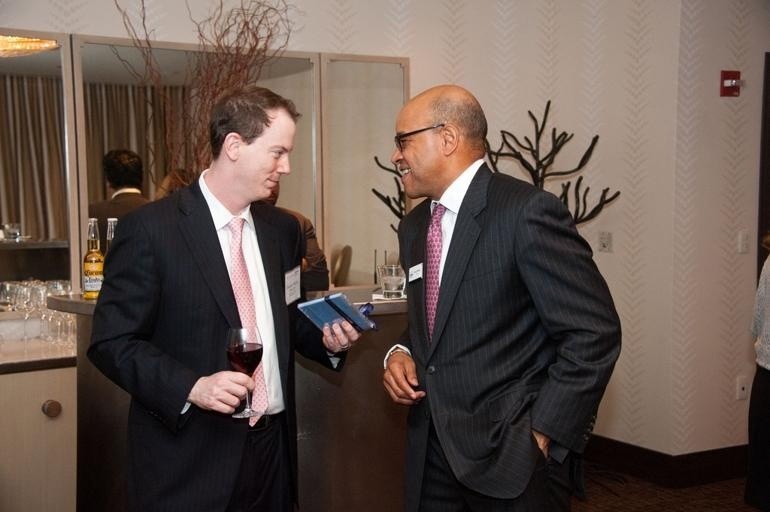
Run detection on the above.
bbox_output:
[341,345,353,352]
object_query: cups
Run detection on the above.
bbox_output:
[4,223,20,244]
[377,264,407,300]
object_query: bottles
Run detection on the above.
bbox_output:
[104,218,119,256]
[83,218,103,303]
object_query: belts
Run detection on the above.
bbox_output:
[246,414,271,433]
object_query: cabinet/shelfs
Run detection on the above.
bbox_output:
[0,358,78,511]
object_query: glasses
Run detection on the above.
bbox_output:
[393,124,444,152]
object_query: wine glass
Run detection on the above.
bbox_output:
[2,279,71,352]
[227,326,262,418]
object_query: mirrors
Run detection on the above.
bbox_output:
[0,27,411,326]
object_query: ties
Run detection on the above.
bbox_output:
[226,220,270,428]
[425,205,447,343]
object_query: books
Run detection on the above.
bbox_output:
[297,292,376,334]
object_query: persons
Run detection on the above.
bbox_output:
[747,236,770,472]
[260,181,330,291]
[86,84,365,512]
[383,83,622,512]
[88,149,154,257]
[153,169,190,200]
